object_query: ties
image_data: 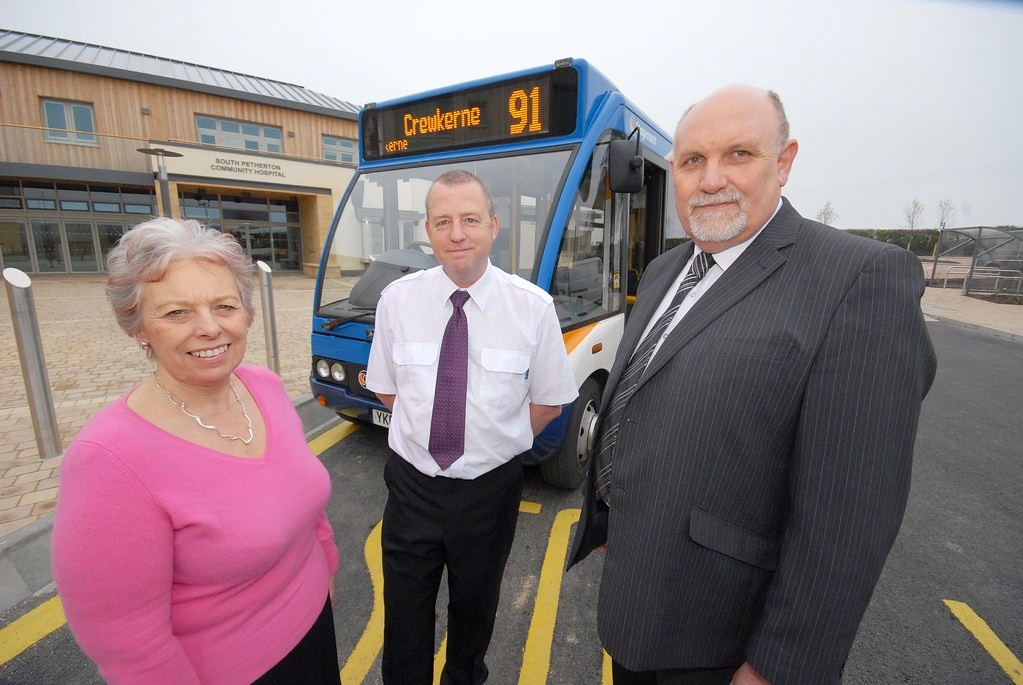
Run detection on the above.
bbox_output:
[592,250,718,503]
[428,290,470,470]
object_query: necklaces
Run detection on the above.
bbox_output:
[152,369,253,445]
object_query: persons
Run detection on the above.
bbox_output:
[564,85,938,685]
[365,170,578,685]
[51,216,343,685]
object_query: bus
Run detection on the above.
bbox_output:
[309,57,690,490]
[209,224,288,260]
